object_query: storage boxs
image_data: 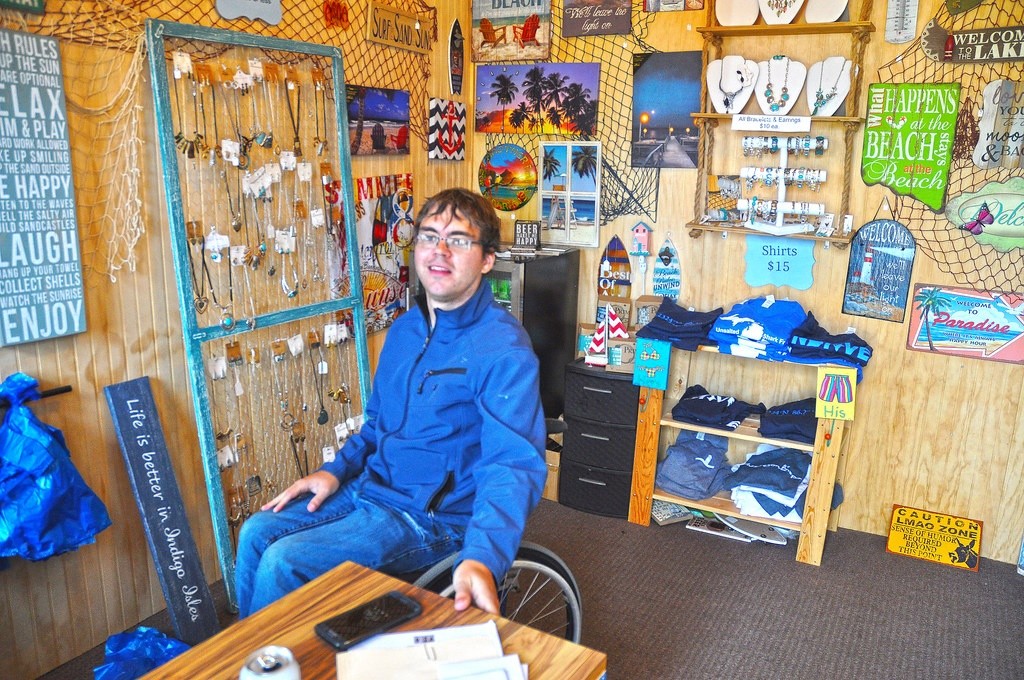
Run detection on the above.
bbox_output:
[576,323,599,361]
[595,295,632,332]
[635,294,665,330]
[605,337,635,374]
[543,433,565,502]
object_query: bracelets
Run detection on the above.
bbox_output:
[719,135,824,225]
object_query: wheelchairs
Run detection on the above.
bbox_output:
[408,539,585,645]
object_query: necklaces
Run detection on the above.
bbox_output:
[223,162,334,297]
[199,77,304,170]
[269,327,351,479]
[173,69,203,159]
[764,56,790,112]
[231,262,255,331]
[202,245,235,331]
[719,57,754,114]
[187,235,209,314]
[313,81,329,155]
[212,368,252,528]
[808,59,848,117]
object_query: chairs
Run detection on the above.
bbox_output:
[368,122,387,155]
[391,125,409,154]
[478,17,507,49]
[513,14,540,49]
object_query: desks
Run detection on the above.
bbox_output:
[134,560,606,679]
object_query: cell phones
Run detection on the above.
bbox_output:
[314,591,422,652]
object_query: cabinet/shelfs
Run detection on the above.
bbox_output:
[687,21,876,243]
[627,336,857,567]
[558,356,638,519]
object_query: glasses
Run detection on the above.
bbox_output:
[416,234,481,251]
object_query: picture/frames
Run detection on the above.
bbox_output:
[539,140,603,247]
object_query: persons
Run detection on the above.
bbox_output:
[451,55,460,70]
[236,186,547,617]
